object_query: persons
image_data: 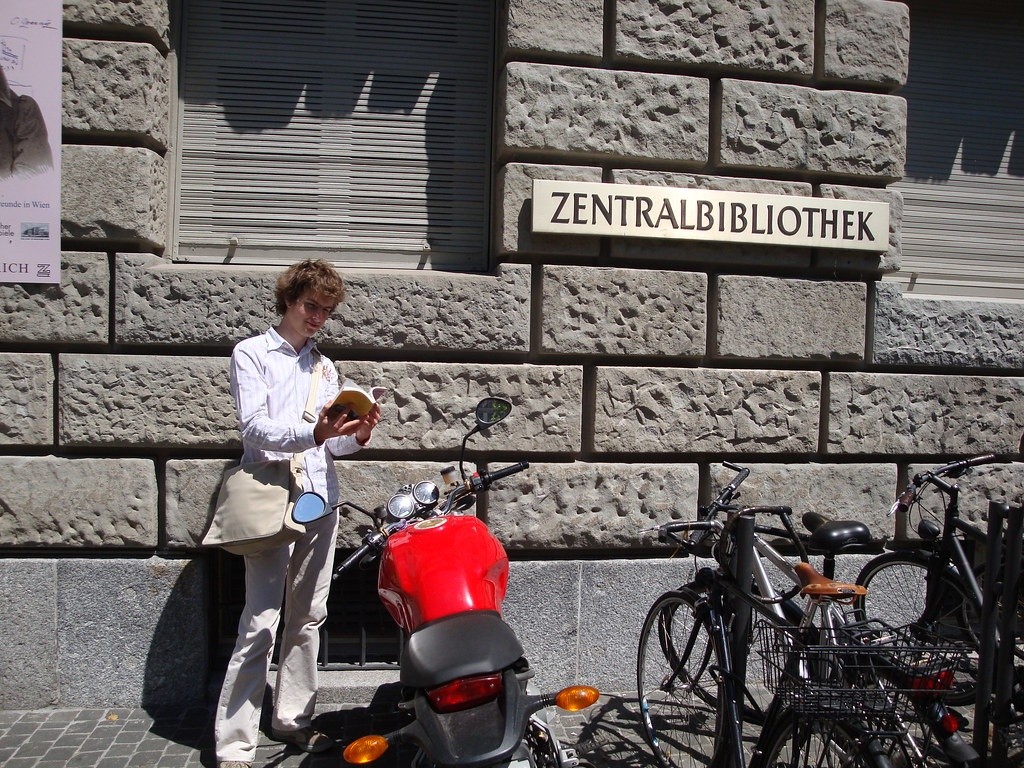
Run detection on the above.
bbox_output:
[214,260,380,768]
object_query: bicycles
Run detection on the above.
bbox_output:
[635,452,1024,768]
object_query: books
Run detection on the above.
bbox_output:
[325,379,387,436]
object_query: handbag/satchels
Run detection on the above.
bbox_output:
[202,459,305,556]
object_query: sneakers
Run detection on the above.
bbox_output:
[272,725,333,753]
[218,760,253,768]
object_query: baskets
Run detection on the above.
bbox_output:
[753,619,975,719]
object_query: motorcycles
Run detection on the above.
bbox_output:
[291,397,600,768]
